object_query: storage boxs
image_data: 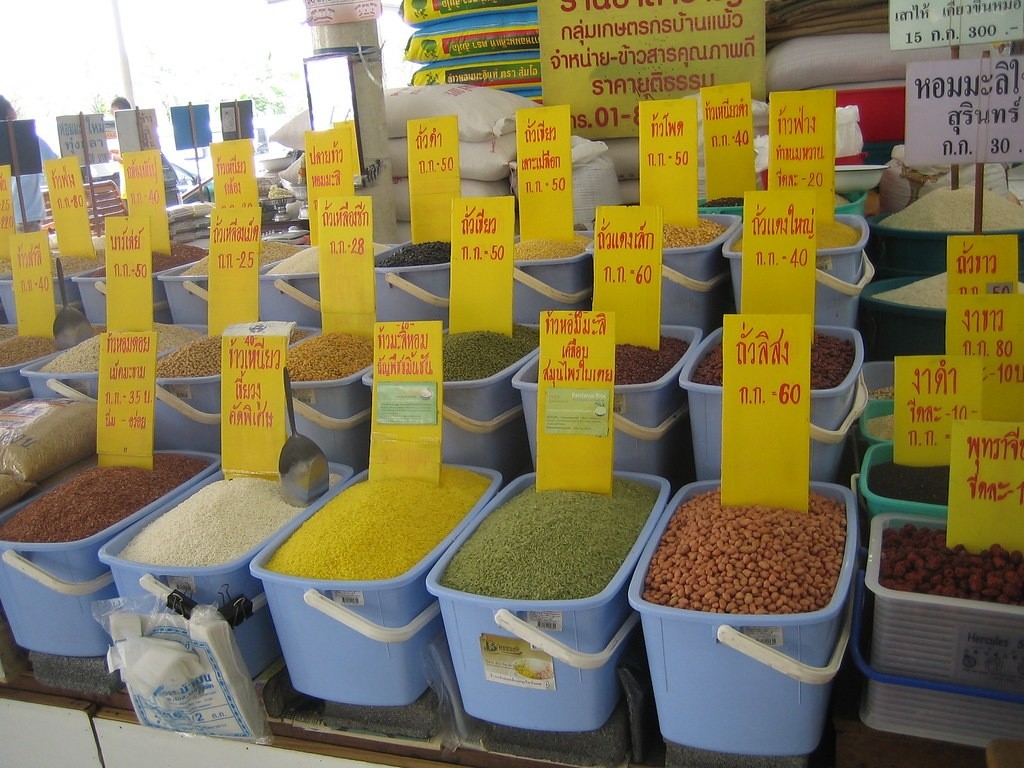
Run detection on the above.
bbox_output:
[754,85,906,190]
[0,191,1024,758]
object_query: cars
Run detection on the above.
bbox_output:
[172,163,215,204]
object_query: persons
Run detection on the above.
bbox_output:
[107,97,132,208]
[0,94,60,234]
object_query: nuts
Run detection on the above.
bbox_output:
[643,486,847,615]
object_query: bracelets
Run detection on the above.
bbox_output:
[112,153,115,162]
